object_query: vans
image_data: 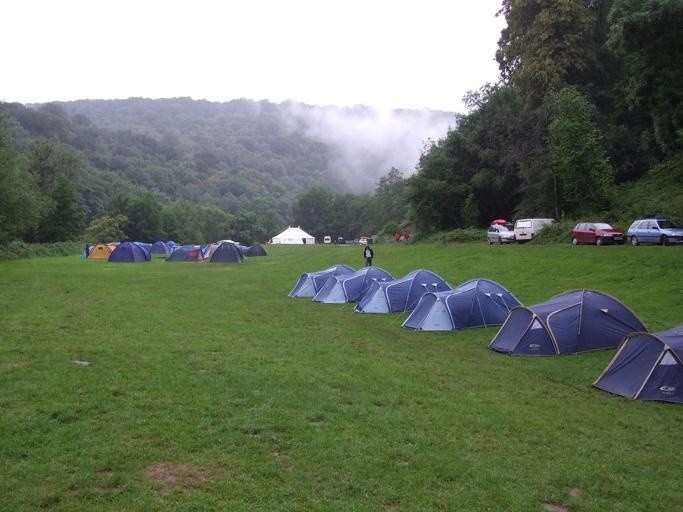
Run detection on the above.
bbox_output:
[323,235,331,244]
[513,218,559,244]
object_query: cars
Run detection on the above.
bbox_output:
[568,219,624,247]
[485,219,515,245]
[625,218,682,247]
[358,236,370,246]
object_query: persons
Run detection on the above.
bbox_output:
[404,231,409,245]
[394,231,401,243]
[364,245,374,267]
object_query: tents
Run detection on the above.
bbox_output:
[311,266,395,305]
[271,224,316,245]
[288,263,357,298]
[488,288,649,357]
[591,324,683,405]
[353,268,453,314]
[80,239,267,262]
[401,278,525,332]
